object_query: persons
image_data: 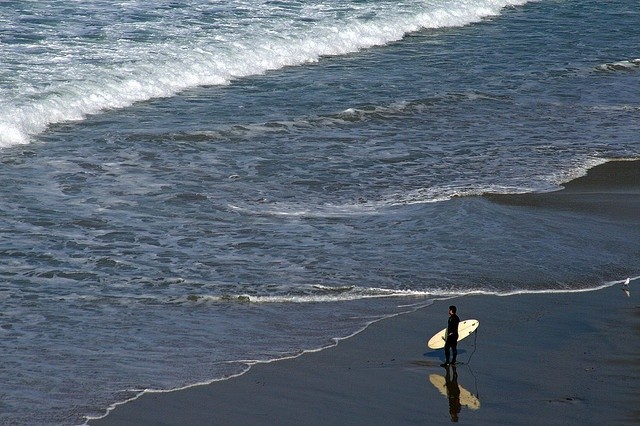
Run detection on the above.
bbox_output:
[441,366,461,422]
[440,306,461,366]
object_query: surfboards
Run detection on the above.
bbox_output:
[428,319,480,350]
[429,373,481,410]
